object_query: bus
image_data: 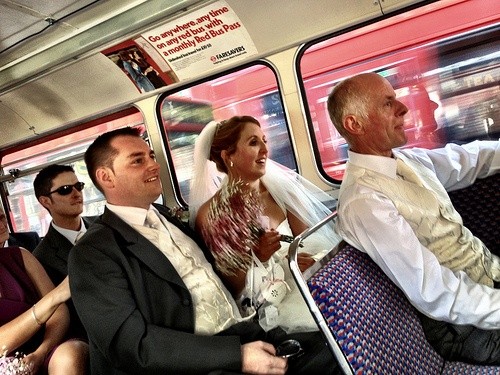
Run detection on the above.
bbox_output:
[0,95,214,241]
[189,1,500,198]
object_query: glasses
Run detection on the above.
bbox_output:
[50,181,85,196]
[270,339,305,362]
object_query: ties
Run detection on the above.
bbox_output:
[146,210,173,240]
[76,232,85,241]
[394,157,424,186]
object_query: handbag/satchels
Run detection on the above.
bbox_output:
[246,248,289,306]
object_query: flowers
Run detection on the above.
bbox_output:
[169,204,189,224]
[0,345,33,375]
[201,173,303,277]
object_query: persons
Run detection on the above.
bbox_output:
[68,128,345,375]
[0,202,90,375]
[28,164,104,345]
[326,72,500,365]
[393,82,440,150]
[190,116,340,332]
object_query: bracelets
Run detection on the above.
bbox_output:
[32,304,42,326]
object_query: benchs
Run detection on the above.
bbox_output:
[288,210,500,375]
